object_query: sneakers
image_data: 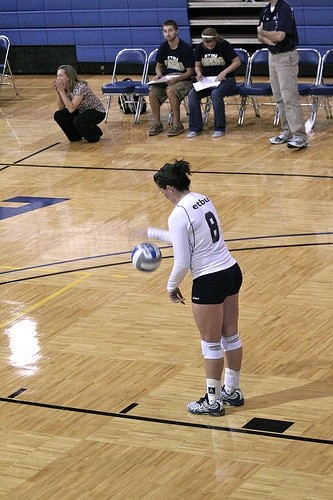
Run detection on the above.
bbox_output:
[187,394,225,415]
[221,385,244,406]
[268,134,292,144]
[287,137,307,148]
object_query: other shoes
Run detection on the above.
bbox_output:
[187,129,202,138]
[213,132,224,138]
[149,123,162,135]
[167,123,184,135]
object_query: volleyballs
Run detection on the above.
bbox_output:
[131,242,163,273]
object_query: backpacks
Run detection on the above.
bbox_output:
[118,78,147,115]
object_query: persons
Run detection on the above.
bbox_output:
[147,158,245,417]
[257,0,308,148]
[52,65,106,143]
[148,20,197,135]
[186,28,242,138]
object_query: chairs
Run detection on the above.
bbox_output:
[101,47,333,131]
[0,34,18,96]
